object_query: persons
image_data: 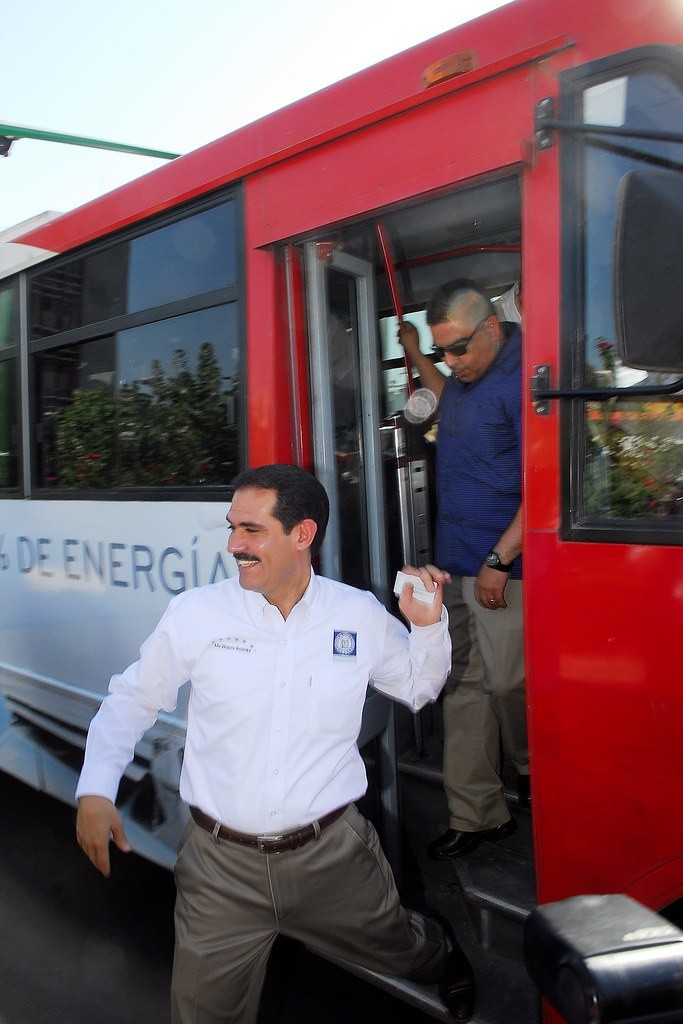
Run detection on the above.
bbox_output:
[397,280,530,857]
[75,464,477,1024]
[491,275,523,322]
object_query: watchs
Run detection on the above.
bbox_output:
[485,550,514,572]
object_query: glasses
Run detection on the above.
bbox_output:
[429,317,487,357]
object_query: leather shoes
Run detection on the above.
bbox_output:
[438,930,475,1023]
[424,816,517,859]
[515,775,531,807]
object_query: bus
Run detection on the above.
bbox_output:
[0,0,683,1024]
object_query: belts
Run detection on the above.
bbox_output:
[189,804,352,855]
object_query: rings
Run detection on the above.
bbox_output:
[488,599,496,603]
